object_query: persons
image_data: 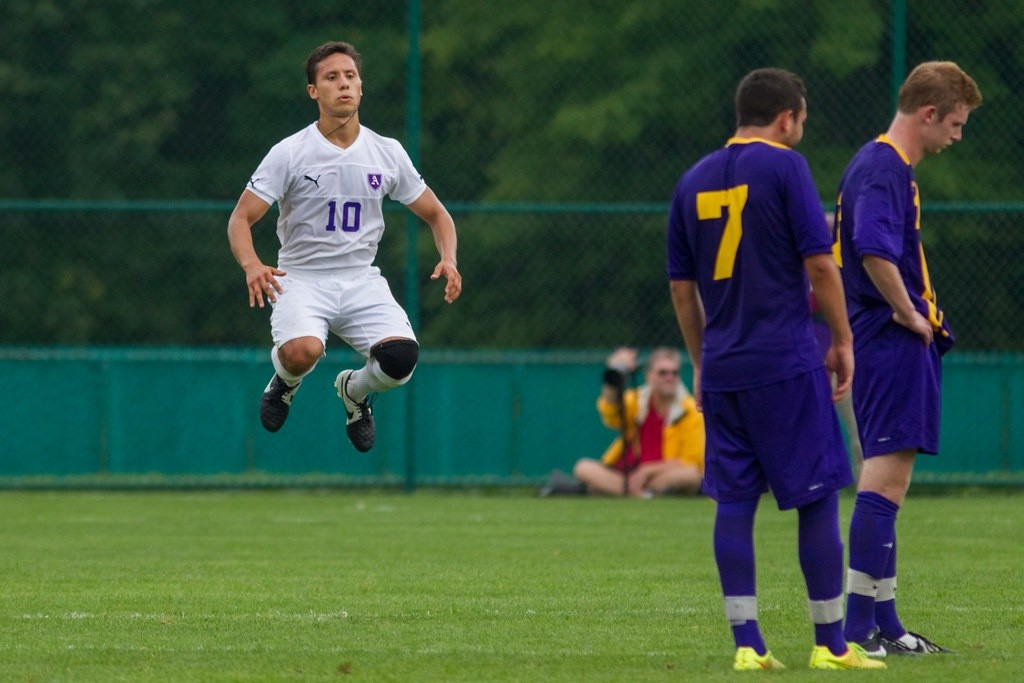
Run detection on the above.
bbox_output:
[227,39,464,454]
[664,67,889,675]
[830,60,983,655]
[537,339,706,498]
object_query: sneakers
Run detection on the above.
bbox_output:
[334,370,376,453]
[857,631,957,659]
[731,647,789,673]
[808,644,888,671]
[259,371,302,433]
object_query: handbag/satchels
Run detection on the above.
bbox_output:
[533,468,588,500]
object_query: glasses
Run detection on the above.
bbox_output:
[651,368,680,377]
